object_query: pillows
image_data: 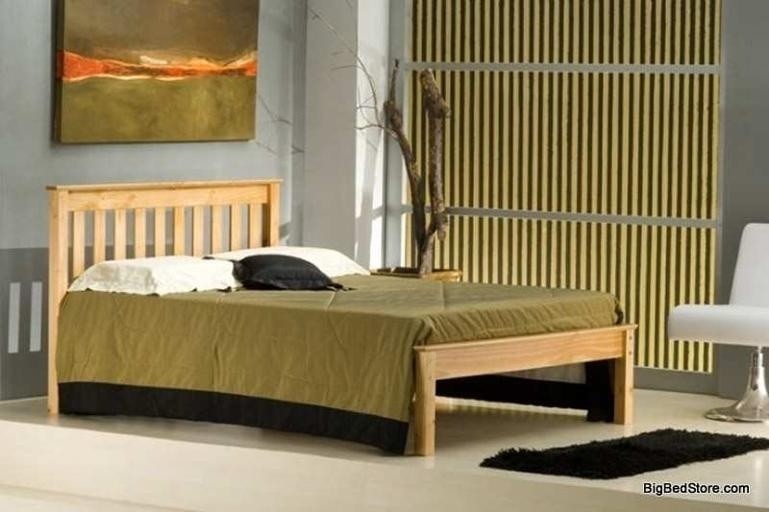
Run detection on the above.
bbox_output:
[66,245,373,305]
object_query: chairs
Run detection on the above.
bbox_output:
[666,222,769,423]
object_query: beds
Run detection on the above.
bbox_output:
[46,178,640,457]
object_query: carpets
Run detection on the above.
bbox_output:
[480,428,768,480]
